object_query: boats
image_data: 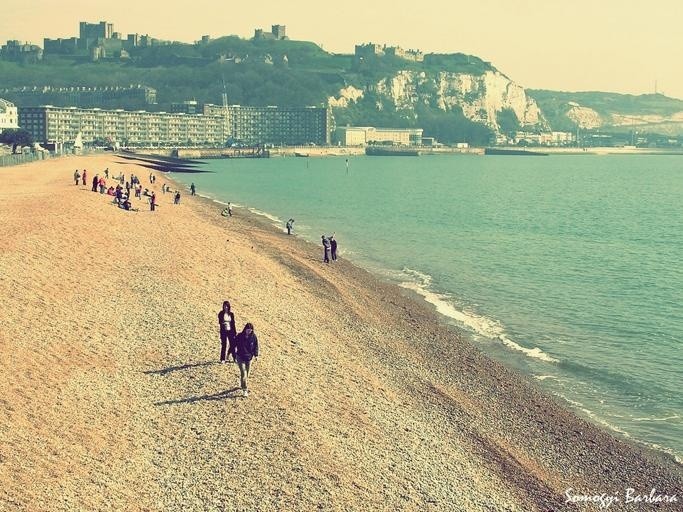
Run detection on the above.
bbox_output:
[365,146,439,156]
[483,148,548,156]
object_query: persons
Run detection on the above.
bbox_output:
[221,202,233,217]
[322,235,337,263]
[74,167,196,211]
[218,301,258,397]
[287,218,294,235]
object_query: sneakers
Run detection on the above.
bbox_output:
[221,360,225,364]
[243,389,248,397]
